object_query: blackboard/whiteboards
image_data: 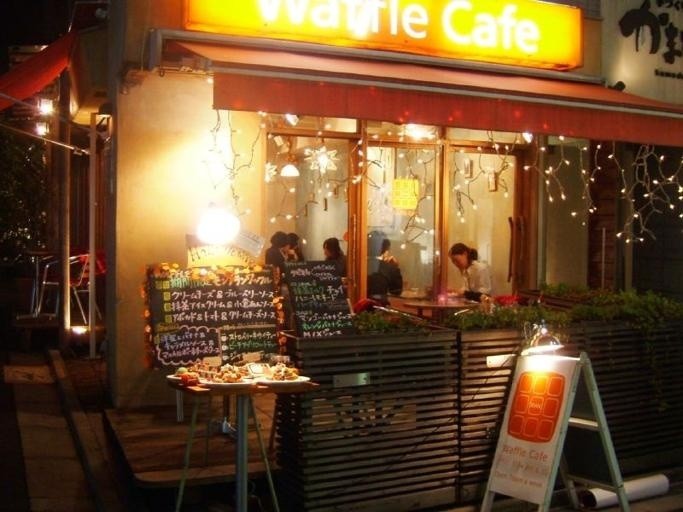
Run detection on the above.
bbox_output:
[148,264,285,369]
[284,259,354,339]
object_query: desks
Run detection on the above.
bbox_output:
[16,247,56,321]
[165,378,320,510]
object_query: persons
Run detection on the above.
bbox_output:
[285,233,304,263]
[446,242,494,296]
[322,237,347,278]
[366,230,402,308]
[263,230,292,266]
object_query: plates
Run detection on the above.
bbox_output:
[164,370,314,388]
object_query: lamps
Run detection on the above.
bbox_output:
[522,320,563,356]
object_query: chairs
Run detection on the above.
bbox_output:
[35,253,103,326]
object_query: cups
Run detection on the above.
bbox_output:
[437,294,447,308]
